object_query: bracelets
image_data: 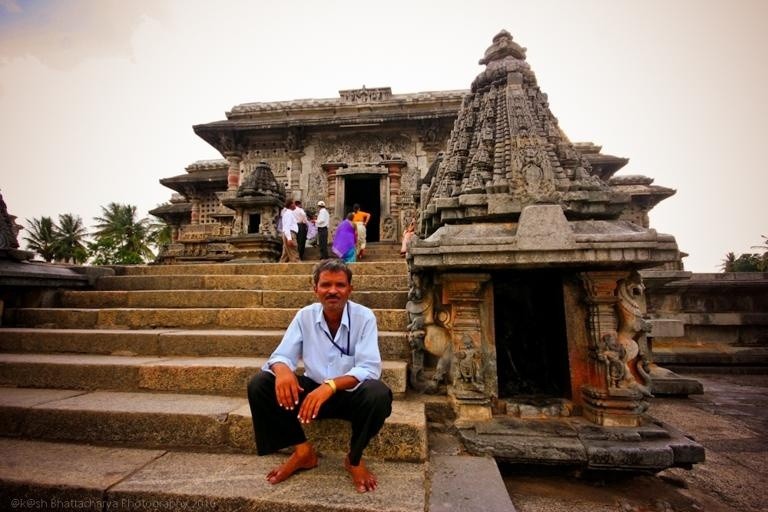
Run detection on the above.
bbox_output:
[323,377,337,396]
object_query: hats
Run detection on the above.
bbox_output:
[317,201,326,207]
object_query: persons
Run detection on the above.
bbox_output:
[245,261,393,495]
[276,198,317,264]
[332,212,361,266]
[350,203,371,262]
[311,201,330,261]
